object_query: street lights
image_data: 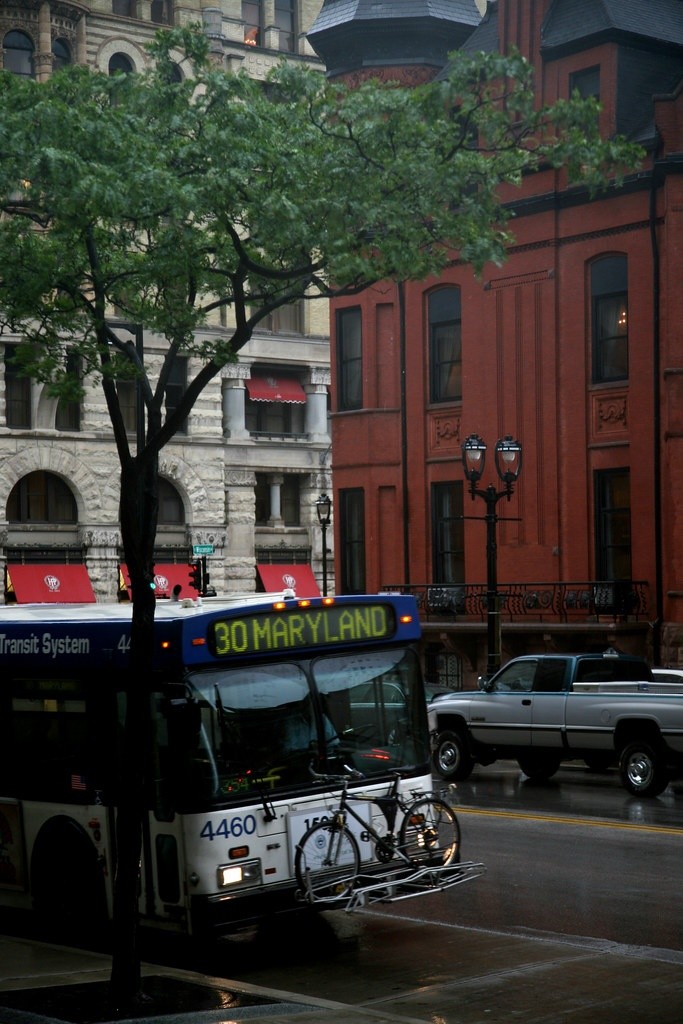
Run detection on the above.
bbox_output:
[461,432,523,691]
[315,492,333,597]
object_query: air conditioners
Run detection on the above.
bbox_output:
[428,588,464,606]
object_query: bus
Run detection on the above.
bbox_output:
[0,584,441,959]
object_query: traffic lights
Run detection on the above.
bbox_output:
[188,560,203,592]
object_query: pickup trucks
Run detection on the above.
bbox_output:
[426,649,683,798]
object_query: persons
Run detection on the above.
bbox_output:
[281,696,342,756]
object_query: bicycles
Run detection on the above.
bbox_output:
[295,764,462,909]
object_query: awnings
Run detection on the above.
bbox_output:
[243,373,307,405]
[8,561,201,604]
[258,564,320,599]
